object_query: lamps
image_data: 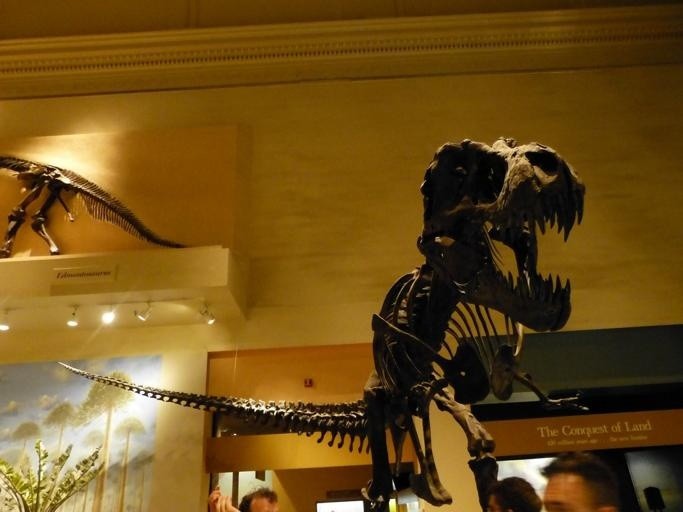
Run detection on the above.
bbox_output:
[0,298,217,332]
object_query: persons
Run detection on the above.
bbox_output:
[486,476,544,512]
[539,451,621,512]
[209,485,278,512]
